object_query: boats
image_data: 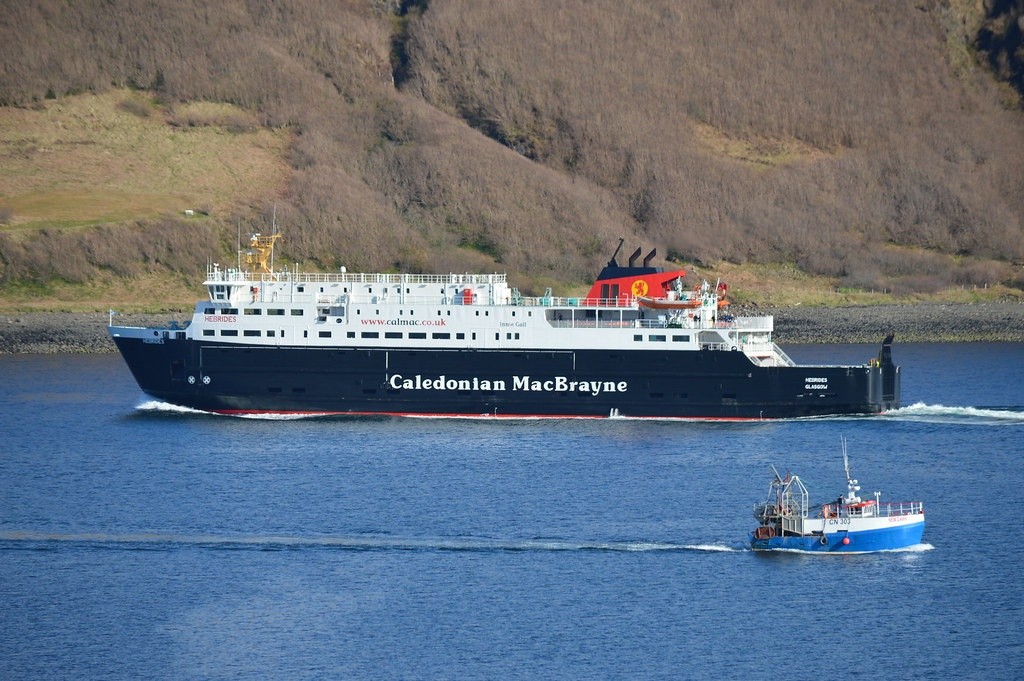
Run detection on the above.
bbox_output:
[106,204,901,421]
[748,435,925,553]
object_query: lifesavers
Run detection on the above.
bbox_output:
[820,537,827,545]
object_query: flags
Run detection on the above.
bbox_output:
[718,280,727,290]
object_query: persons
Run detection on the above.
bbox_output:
[558,313,563,320]
